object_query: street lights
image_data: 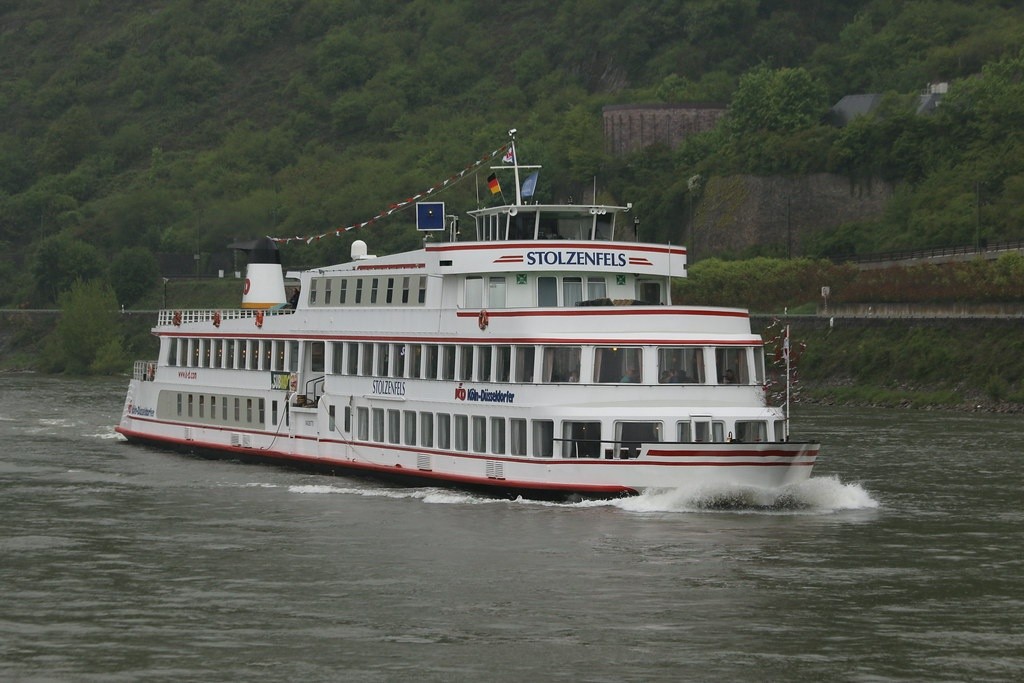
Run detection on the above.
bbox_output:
[634,215,640,242]
[162,277,168,308]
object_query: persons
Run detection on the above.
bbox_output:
[658,369,688,383]
[725,369,736,384]
[620,368,634,383]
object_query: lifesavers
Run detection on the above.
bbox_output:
[477,309,489,331]
[255,311,263,328]
[213,311,221,328]
[173,311,180,326]
[146,365,153,379]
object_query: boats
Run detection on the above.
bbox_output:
[115,130,822,499]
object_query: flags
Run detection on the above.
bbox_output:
[520,172,537,196]
[487,173,500,194]
[265,144,508,245]
[502,148,513,163]
